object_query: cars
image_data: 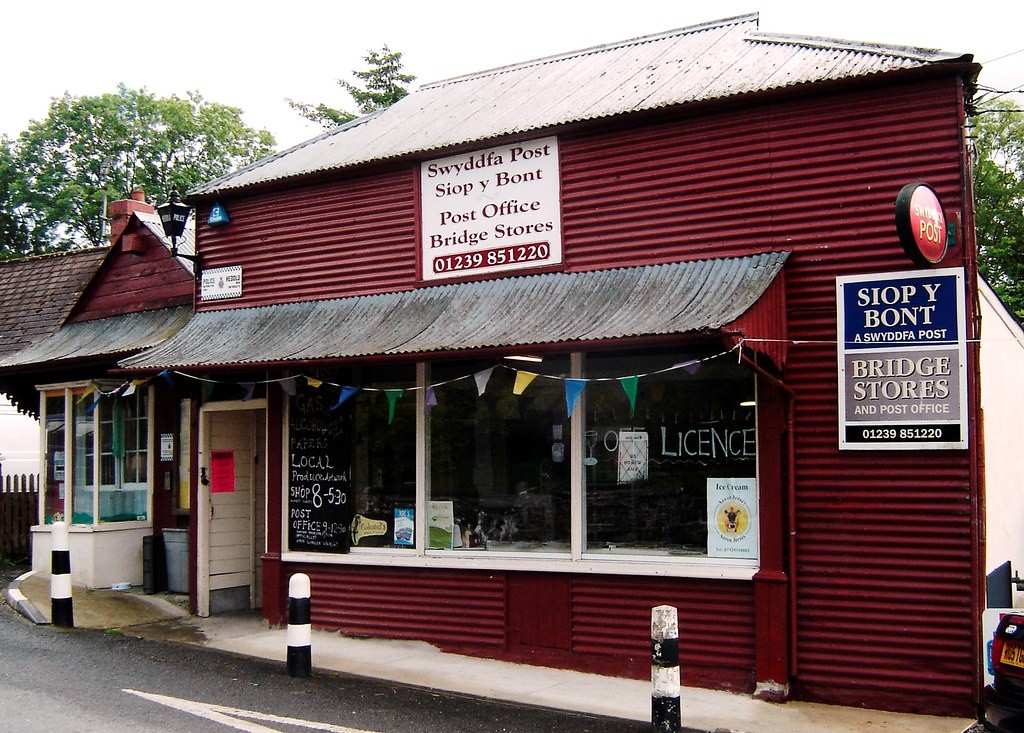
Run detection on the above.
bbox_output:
[974,610,1024,733]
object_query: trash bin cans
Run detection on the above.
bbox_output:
[161,528,190,594]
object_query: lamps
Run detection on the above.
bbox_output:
[156,182,202,280]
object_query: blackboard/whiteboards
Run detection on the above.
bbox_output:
[289,384,353,551]
